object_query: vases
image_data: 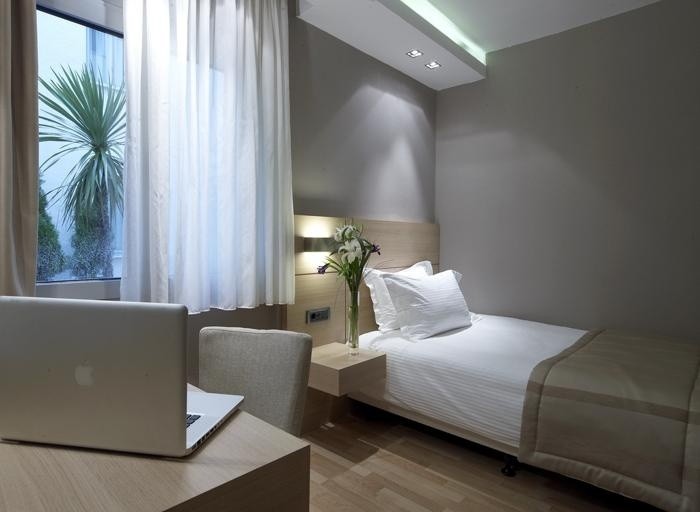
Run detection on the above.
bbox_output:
[348,290,359,354]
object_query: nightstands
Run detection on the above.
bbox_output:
[306,341,386,398]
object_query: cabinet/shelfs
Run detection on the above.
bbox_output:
[1,382,310,512]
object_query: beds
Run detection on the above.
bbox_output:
[282,214,700,512]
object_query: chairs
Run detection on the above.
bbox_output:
[198,325,312,438]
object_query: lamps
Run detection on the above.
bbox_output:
[304,237,334,253]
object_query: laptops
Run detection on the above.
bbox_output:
[0,296,245,458]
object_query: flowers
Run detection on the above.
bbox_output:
[318,216,381,341]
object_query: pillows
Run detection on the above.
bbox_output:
[379,269,481,343]
[362,260,434,334]
